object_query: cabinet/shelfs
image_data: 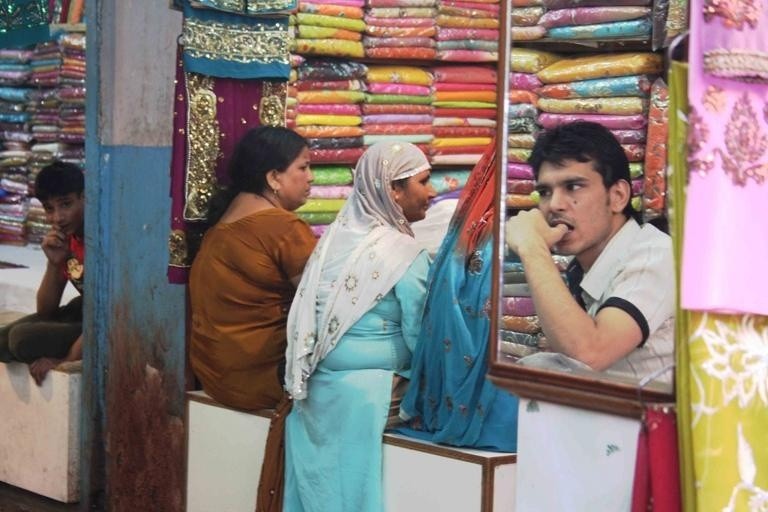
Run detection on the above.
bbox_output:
[183,390,521,512]
[169,0,500,387]
[0,355,90,506]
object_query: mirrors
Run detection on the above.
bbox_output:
[486,0,687,419]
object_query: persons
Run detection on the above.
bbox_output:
[188,126,321,414]
[398,138,518,454]
[503,118,674,385]
[0,161,90,384]
[287,140,434,430]
